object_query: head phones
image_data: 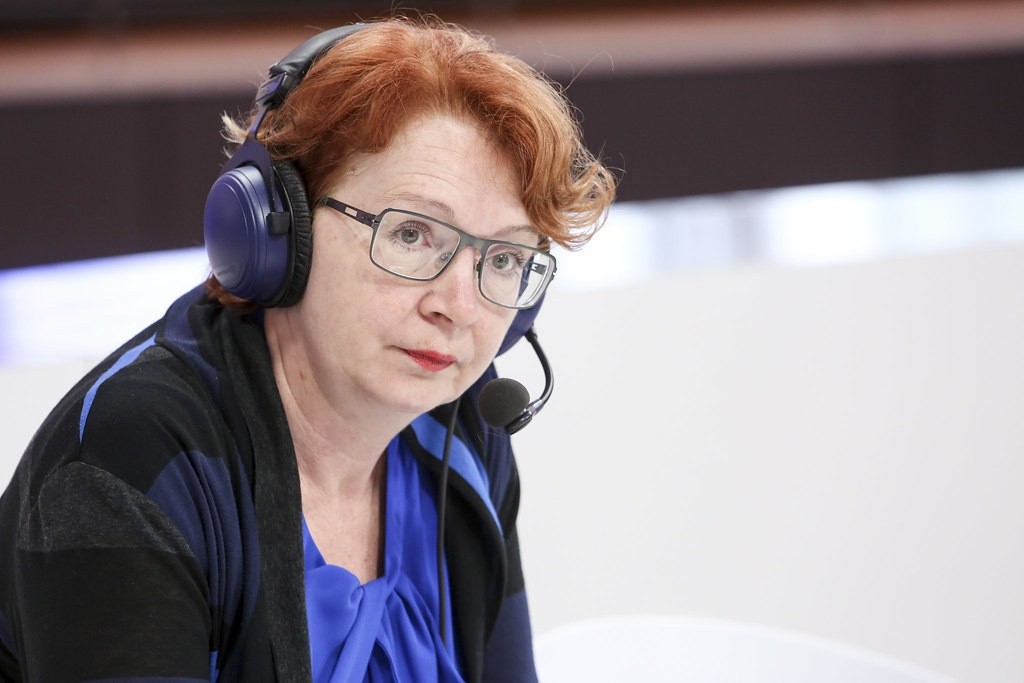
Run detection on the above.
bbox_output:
[202,22,548,360]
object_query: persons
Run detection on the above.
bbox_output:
[1,22,614,683]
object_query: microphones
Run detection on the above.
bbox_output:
[477,336,554,435]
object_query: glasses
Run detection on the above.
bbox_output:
[317,196,557,310]
